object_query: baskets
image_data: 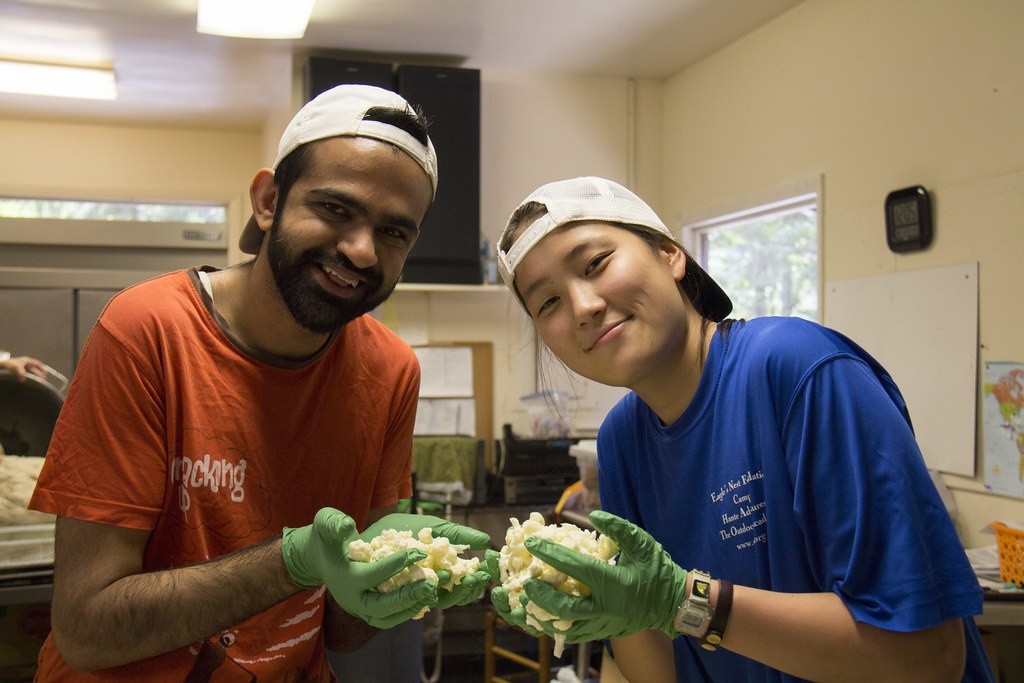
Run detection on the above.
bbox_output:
[990,519,1024,589]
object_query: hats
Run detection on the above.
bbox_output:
[239,84,438,254]
[495,176,733,323]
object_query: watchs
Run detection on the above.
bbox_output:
[671,567,713,638]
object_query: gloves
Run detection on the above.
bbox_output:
[483,548,544,636]
[520,509,690,644]
[282,506,440,629]
[360,512,489,608]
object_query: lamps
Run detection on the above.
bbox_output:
[195,0,314,40]
[0,57,118,102]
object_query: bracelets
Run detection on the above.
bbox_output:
[698,578,734,653]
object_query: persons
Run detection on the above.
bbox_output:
[495,177,996,683]
[0,350,48,382]
[26,85,500,683]
